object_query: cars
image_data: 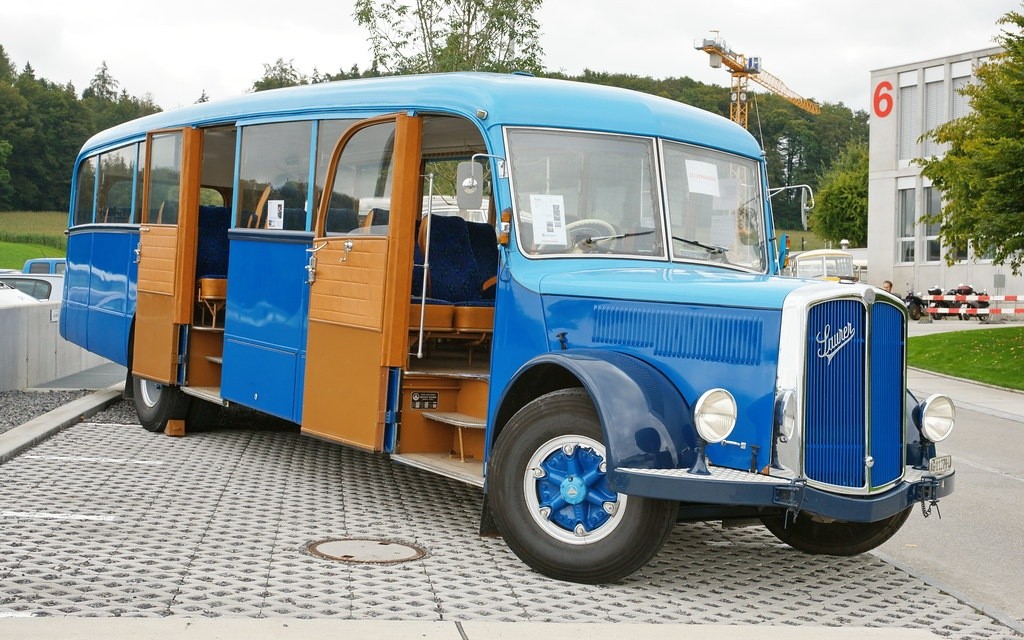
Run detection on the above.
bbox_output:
[781,238,861,283]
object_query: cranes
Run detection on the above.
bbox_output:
[697,33,819,131]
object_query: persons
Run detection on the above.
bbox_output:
[883,281,893,292]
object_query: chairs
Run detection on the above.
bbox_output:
[107,201,495,365]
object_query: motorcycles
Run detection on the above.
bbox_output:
[906,283,991,321]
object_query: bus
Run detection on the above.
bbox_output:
[56,72,958,589]
